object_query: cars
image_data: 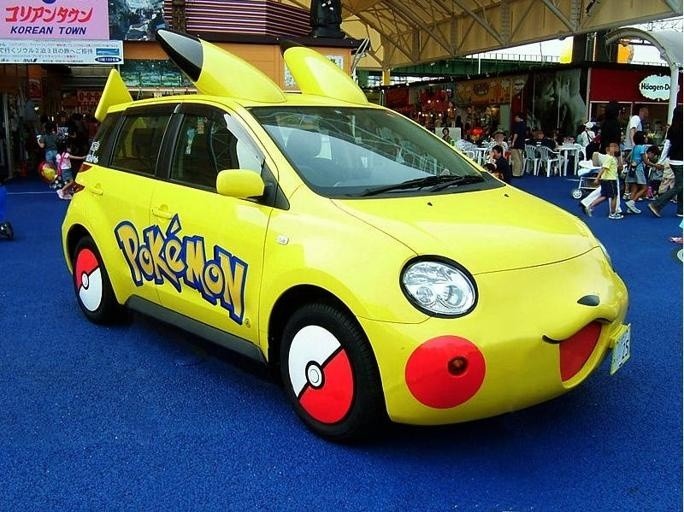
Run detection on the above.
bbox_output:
[55,25,634,439]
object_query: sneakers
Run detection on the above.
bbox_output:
[585,204,592,217]
[625,200,641,214]
[57,189,72,200]
[608,213,624,219]
[647,202,662,218]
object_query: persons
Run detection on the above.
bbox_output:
[521,71,556,141]
[36,111,85,199]
[419,97,683,247]
[553,68,586,146]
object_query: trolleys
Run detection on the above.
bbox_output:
[568,145,631,201]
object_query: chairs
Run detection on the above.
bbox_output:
[132,127,164,176]
[285,128,344,188]
[360,129,591,179]
